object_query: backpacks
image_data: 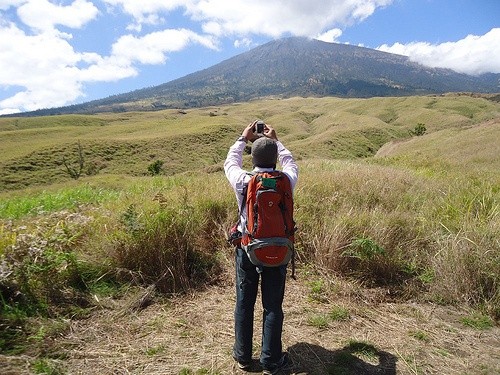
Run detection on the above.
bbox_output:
[229,170,300,282]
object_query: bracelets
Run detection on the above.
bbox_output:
[237,136,248,144]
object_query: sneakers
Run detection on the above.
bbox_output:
[232,356,252,369]
[261,352,290,375]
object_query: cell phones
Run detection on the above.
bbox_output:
[256,121,265,136]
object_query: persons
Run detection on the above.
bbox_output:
[222,120,298,375]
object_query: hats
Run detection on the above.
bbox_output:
[251,136,279,165]
